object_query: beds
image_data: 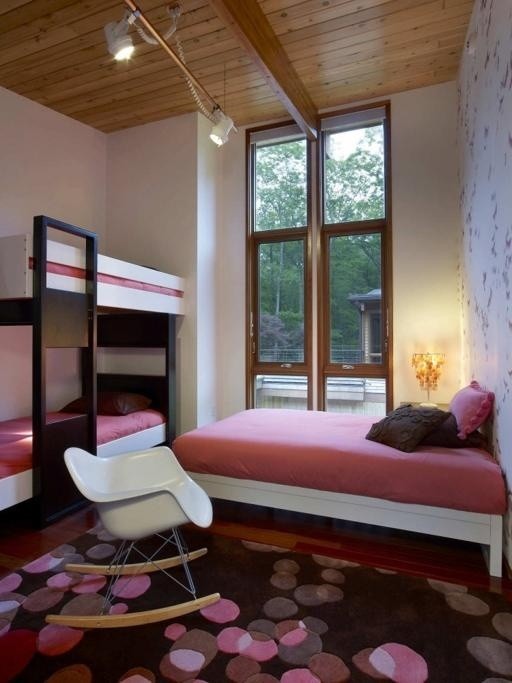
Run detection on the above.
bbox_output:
[173,408,508,578]
[0,216,186,527]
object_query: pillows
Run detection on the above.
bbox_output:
[421,414,488,448]
[365,401,452,453]
[62,391,153,414]
[449,380,494,440]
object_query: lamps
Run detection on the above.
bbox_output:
[103,0,239,148]
[411,345,448,408]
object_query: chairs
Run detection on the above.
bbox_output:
[44,444,221,626]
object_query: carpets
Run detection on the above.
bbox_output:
[0,521,512,683]
